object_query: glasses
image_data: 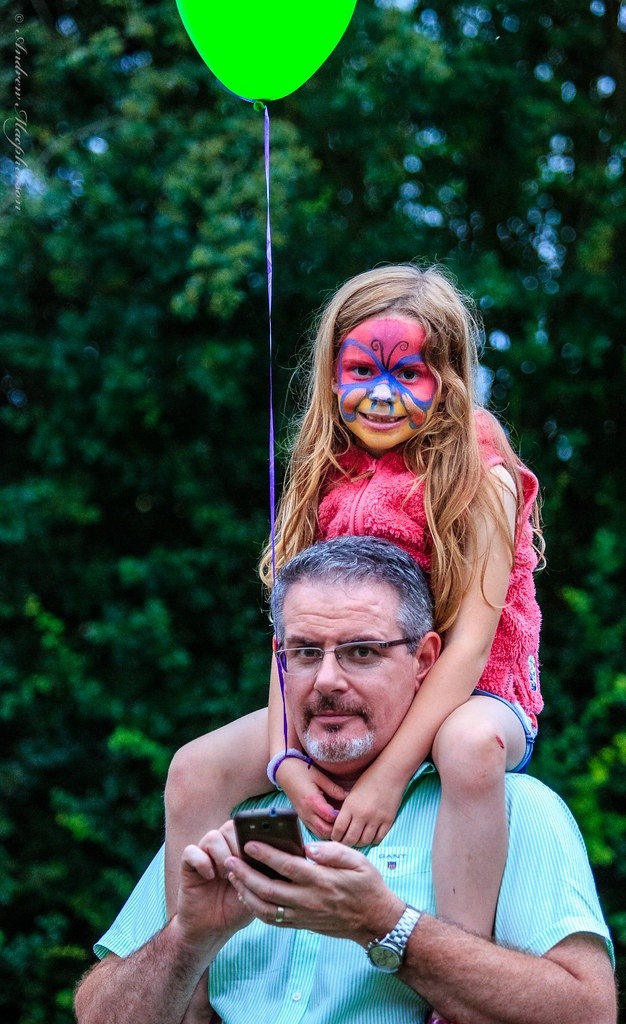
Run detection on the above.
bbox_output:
[273,630,434,675]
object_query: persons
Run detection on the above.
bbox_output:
[74,264,617,1024]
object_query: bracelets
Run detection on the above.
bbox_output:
[267,748,306,785]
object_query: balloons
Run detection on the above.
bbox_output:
[176,0,356,113]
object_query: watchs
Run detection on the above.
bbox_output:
[366,905,422,974]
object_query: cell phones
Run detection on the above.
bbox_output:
[232,807,306,884]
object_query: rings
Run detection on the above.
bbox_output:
[275,906,284,923]
